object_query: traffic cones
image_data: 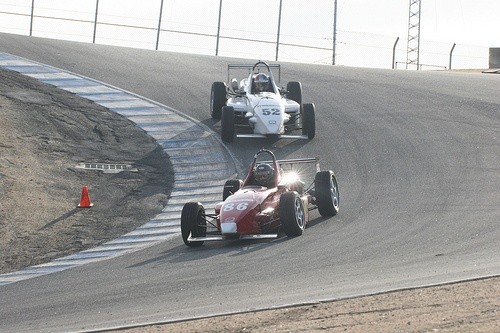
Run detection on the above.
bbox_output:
[78,185,95,208]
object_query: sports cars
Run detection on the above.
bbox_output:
[207,61,319,142]
[179,149,341,244]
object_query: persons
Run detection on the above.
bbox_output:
[251,164,276,190]
[254,74,269,93]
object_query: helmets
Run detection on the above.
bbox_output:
[253,73,269,91]
[254,163,275,186]
